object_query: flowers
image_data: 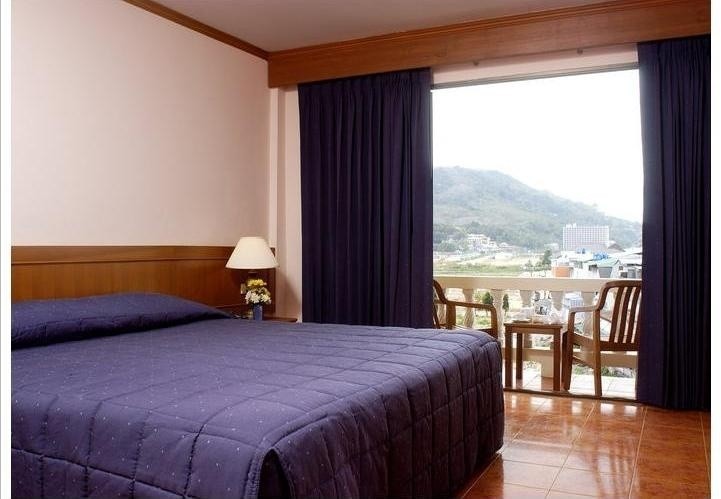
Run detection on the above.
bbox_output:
[244,279,271,305]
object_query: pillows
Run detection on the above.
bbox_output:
[12,294,232,345]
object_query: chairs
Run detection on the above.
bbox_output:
[433,279,498,339]
[565,279,642,396]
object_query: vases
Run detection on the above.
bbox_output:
[253,305,262,321]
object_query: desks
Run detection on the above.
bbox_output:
[502,321,568,392]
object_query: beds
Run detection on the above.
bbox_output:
[12,294,503,498]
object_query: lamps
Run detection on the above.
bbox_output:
[224,236,280,278]
